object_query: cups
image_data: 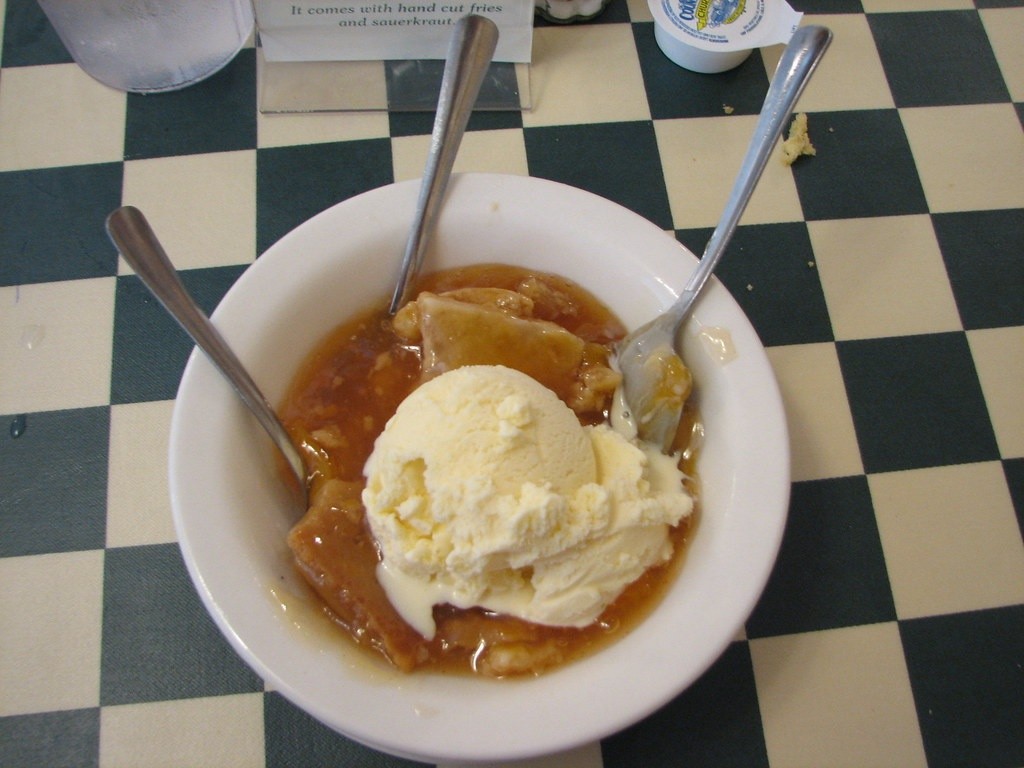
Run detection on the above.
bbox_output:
[38,0,255,95]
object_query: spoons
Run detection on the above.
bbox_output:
[619,23,835,452]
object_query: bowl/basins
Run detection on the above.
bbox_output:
[165,171,794,765]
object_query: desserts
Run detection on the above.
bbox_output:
[357,360,695,638]
